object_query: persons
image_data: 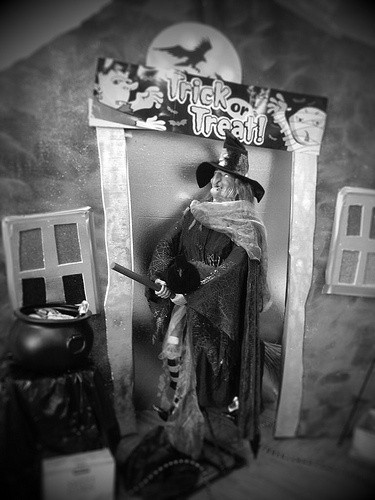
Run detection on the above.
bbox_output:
[144,129,269,464]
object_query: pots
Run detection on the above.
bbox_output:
[9,303,95,373]
